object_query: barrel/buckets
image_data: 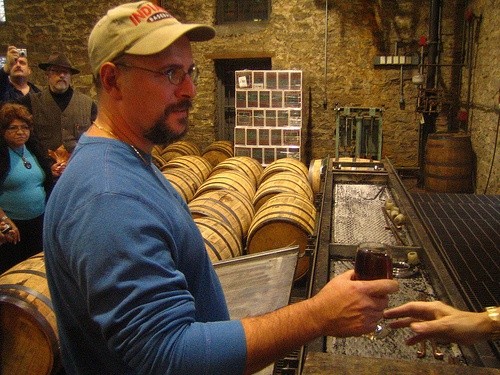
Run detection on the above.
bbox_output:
[260,153,307,187]
[147,142,165,166]
[194,170,253,205]
[158,167,199,201]
[246,193,316,281]
[186,189,254,244]
[200,141,234,165]
[208,155,261,182]
[193,216,242,265]
[253,172,313,213]
[167,155,212,179]
[425,130,472,194]
[308,157,385,196]
[159,140,202,161]
[0,247,58,375]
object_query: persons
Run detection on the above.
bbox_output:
[42,0,400,375]
[20,55,99,201]
[383,300,500,347]
[0,101,67,246]
[0,45,42,114]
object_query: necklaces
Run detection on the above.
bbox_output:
[9,145,32,169]
[90,119,151,167]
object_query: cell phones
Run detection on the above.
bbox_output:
[1,224,12,233]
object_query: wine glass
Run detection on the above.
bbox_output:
[352,242,392,339]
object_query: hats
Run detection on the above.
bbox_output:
[87,1,216,80]
[38,55,80,75]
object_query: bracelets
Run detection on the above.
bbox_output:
[484,306,500,338]
[1,215,8,221]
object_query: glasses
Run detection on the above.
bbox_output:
[114,62,200,86]
[7,125,31,132]
[48,70,68,75]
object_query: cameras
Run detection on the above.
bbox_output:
[17,49,27,58]
